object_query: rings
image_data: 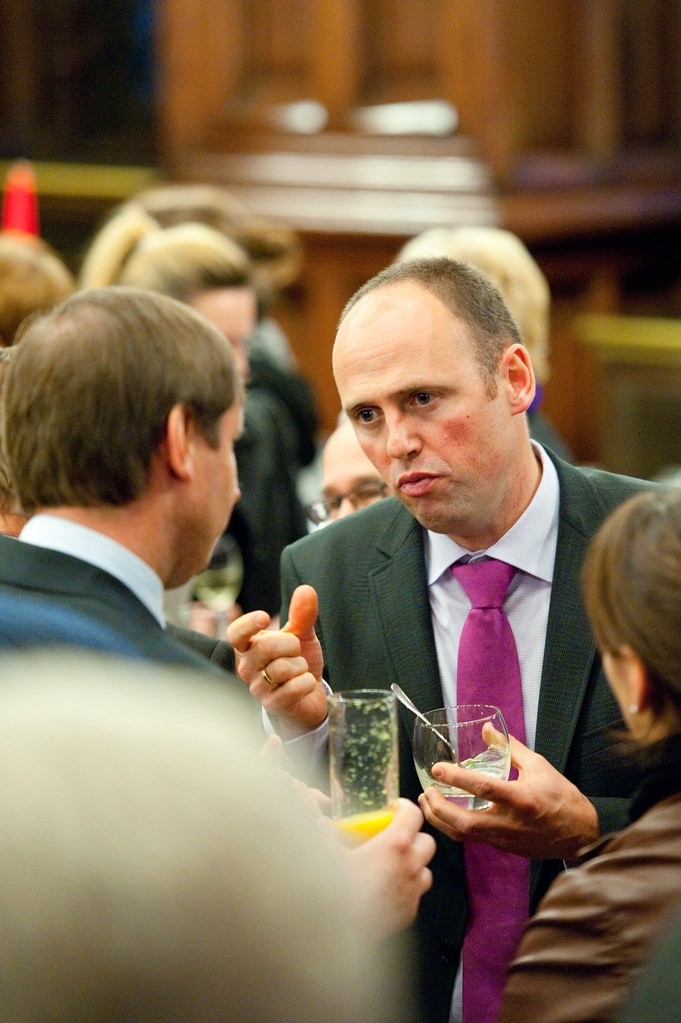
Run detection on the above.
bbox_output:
[232,646,246,658]
[261,670,277,690]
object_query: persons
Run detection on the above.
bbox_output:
[224,253,668,1022]
[0,646,415,1022]
[495,473,681,1023]
[124,182,320,465]
[80,204,313,635]
[1,288,436,936]
[320,423,396,527]
[1,234,76,364]
[387,228,581,472]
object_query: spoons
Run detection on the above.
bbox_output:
[391,680,467,772]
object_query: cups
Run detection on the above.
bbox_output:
[328,689,399,849]
[412,705,511,814]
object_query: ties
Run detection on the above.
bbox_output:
[447,558,531,1023]
